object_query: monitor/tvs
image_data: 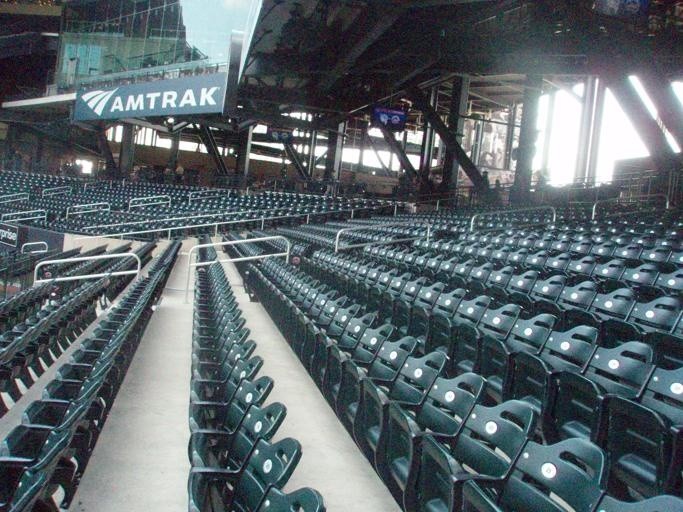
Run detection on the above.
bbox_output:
[372,106,407,131]
[267,125,292,142]
